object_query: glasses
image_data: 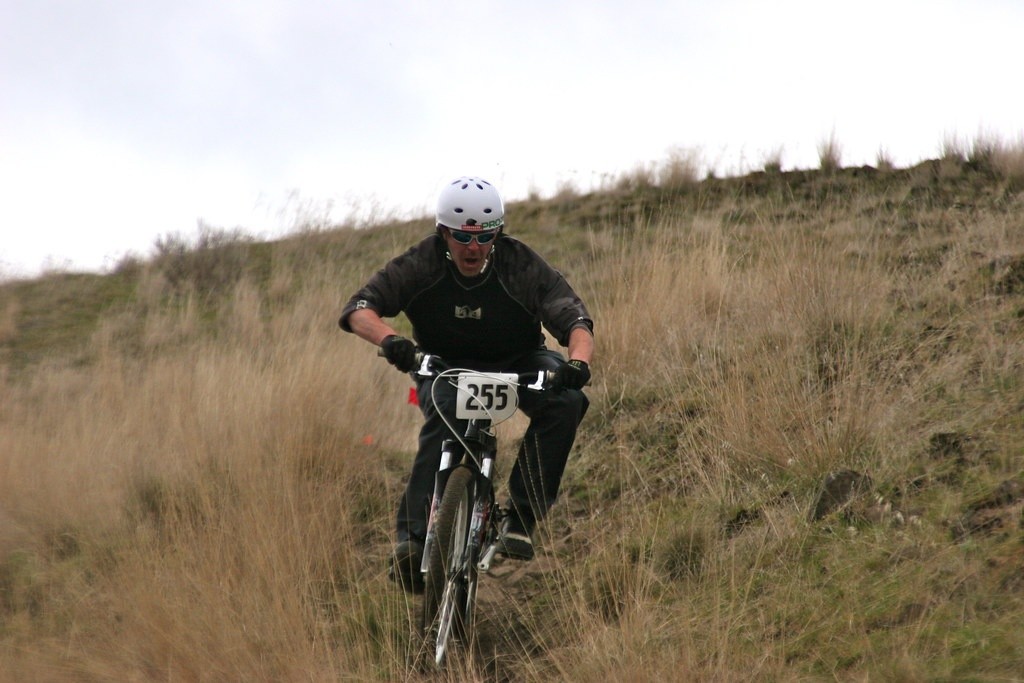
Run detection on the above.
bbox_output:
[446,227,501,245]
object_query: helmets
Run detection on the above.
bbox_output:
[434,175,504,238]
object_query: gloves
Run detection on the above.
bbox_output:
[553,359,591,392]
[380,334,416,374]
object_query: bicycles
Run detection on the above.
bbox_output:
[377,345,593,674]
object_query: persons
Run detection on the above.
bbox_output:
[337,177,595,596]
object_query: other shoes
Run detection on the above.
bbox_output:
[497,504,538,560]
[388,538,423,587]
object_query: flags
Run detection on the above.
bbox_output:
[407,387,418,406]
